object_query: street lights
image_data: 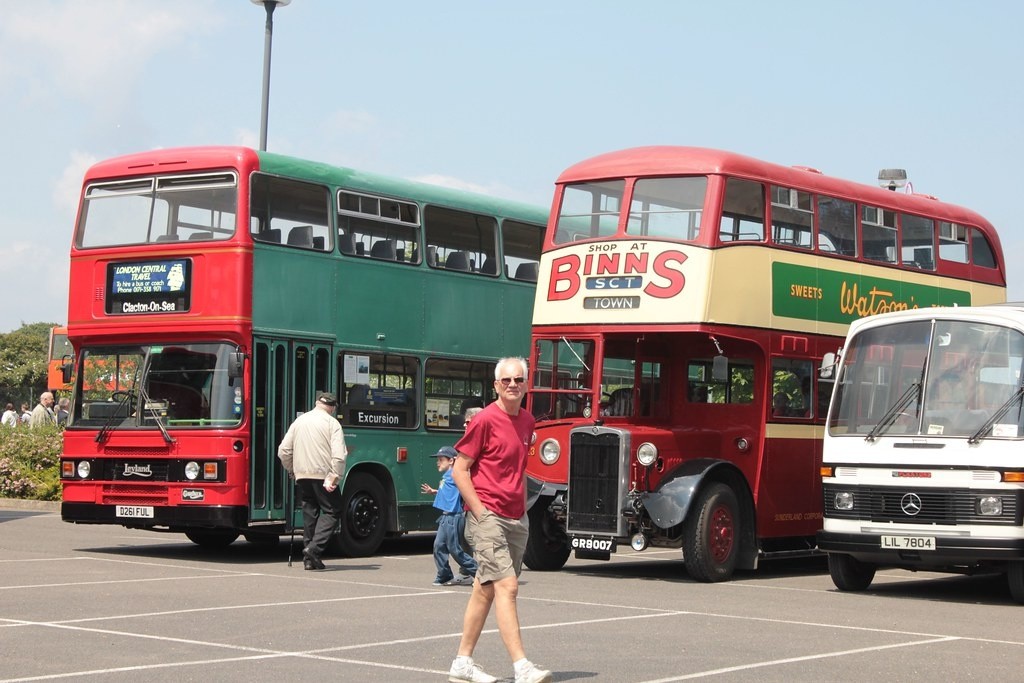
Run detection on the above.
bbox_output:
[250,0,292,149]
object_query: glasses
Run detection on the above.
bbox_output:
[496,378,524,385]
[463,421,470,425]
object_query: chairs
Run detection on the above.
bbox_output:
[773,392,792,417]
[188,232,213,240]
[348,384,484,428]
[260,226,539,281]
[604,387,636,417]
[155,235,179,241]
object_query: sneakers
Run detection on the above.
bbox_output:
[514,661,552,683]
[449,657,497,683]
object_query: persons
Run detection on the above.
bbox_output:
[448,356,553,683]
[279,392,348,570]
[1,391,70,429]
[773,392,791,417]
[420,407,483,585]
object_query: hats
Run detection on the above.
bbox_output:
[318,393,338,408]
[429,446,457,458]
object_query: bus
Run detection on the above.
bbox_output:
[61,145,709,574]
[520,146,1007,582]
[817,301,1024,607]
[46,324,142,410]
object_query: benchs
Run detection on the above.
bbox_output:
[923,406,1024,433]
[718,233,924,269]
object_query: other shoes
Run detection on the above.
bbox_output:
[305,560,314,570]
[447,572,473,584]
[303,548,324,569]
[432,576,455,585]
